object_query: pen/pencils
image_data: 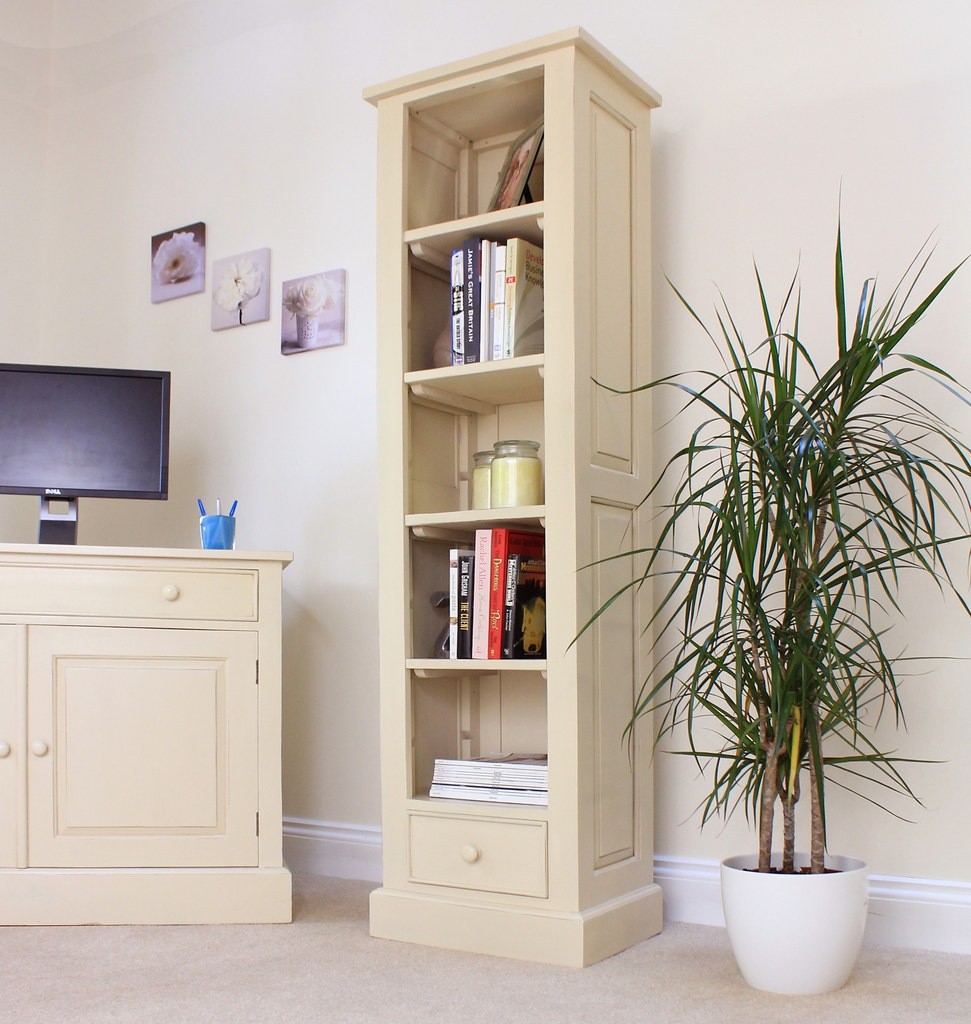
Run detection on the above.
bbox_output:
[229,499,238,517]
[197,498,206,517]
[217,498,221,516]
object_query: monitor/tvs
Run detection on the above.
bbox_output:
[0,362,172,544]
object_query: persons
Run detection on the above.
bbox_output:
[498,146,533,209]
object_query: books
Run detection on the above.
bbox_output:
[427,752,548,806]
[449,237,544,366]
[449,526,547,662]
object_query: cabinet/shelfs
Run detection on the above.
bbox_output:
[0,542,295,923]
[359,23,663,971]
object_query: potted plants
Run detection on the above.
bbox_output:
[560,168,971,995]
